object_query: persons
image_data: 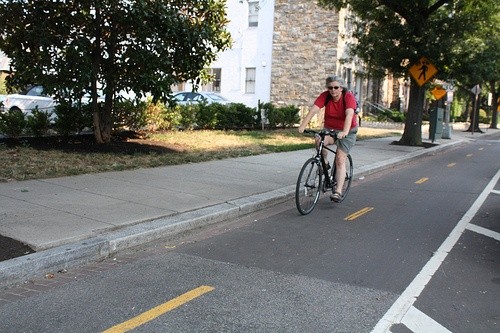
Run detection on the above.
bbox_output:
[298,76,358,201]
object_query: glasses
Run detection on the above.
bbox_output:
[328,86,339,89]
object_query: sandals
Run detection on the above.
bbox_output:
[316,162,331,175]
[330,192,341,201]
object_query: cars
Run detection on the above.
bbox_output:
[3,82,105,123]
[168,91,234,106]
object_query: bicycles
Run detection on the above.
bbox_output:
[295,129,353,215]
